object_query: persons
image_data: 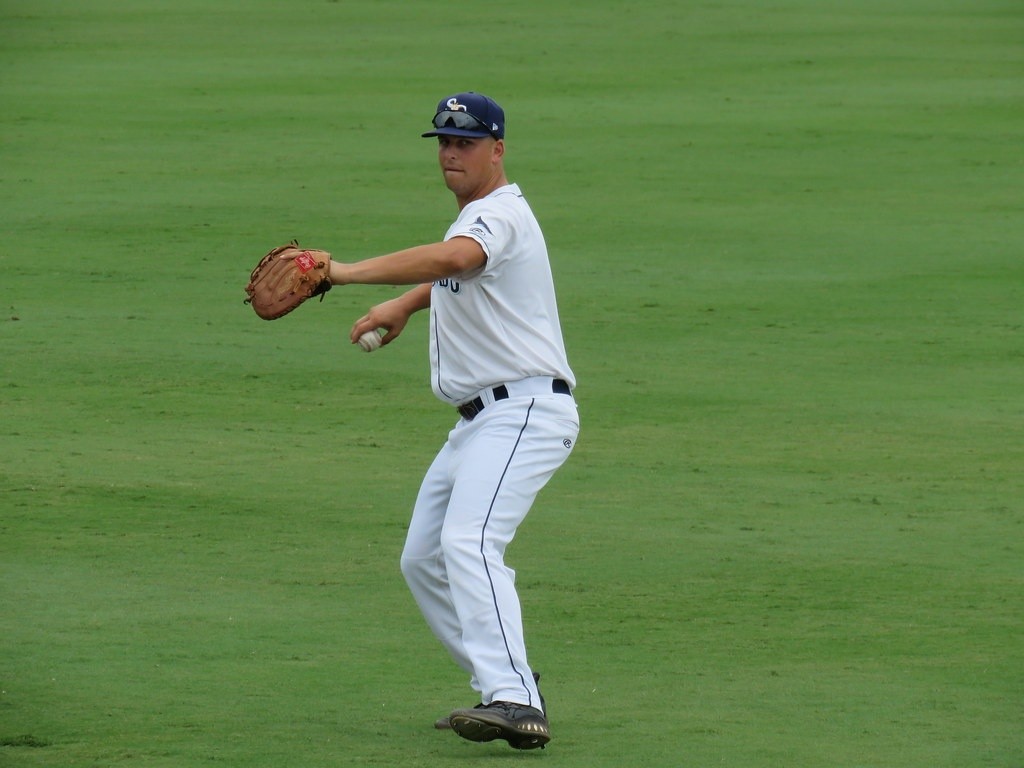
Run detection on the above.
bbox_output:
[280,91,581,751]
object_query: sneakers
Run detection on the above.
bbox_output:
[449,701,551,750]
[434,672,546,729]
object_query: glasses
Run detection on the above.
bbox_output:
[432,110,500,141]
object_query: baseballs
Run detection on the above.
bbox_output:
[356,330,382,353]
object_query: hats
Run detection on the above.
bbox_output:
[421,92,505,140]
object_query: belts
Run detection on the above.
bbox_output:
[457,379,571,422]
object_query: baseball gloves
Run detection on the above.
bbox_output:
[244,245,332,321]
